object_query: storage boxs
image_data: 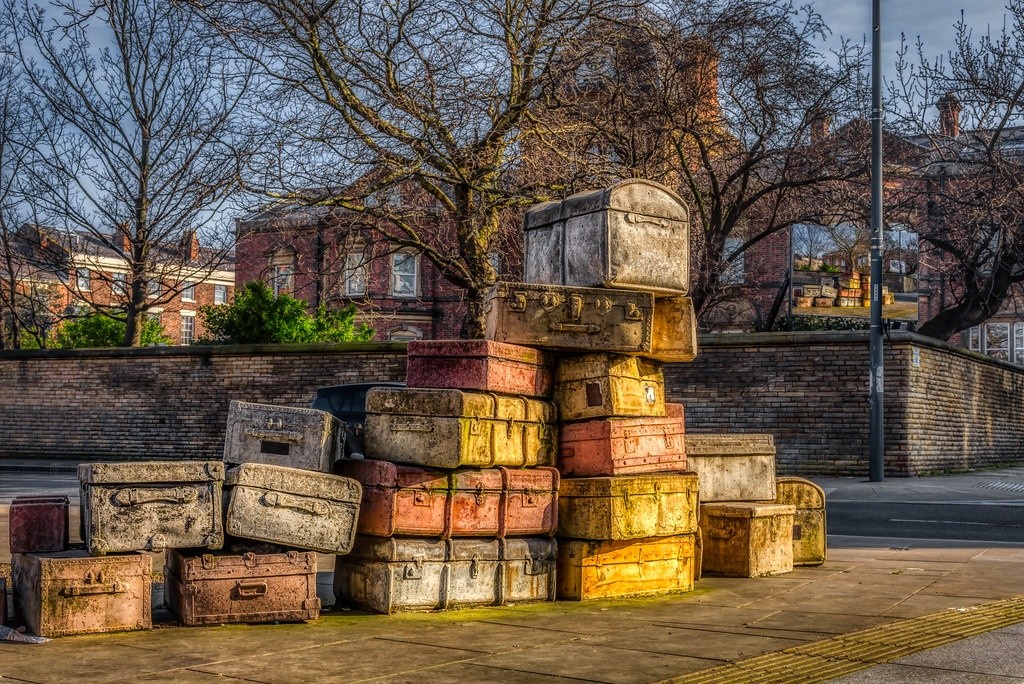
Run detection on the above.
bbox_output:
[522,177,689,298]
[341,338,829,613]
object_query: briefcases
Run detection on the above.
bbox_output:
[7,178,825,636]
[796,269,895,309]
